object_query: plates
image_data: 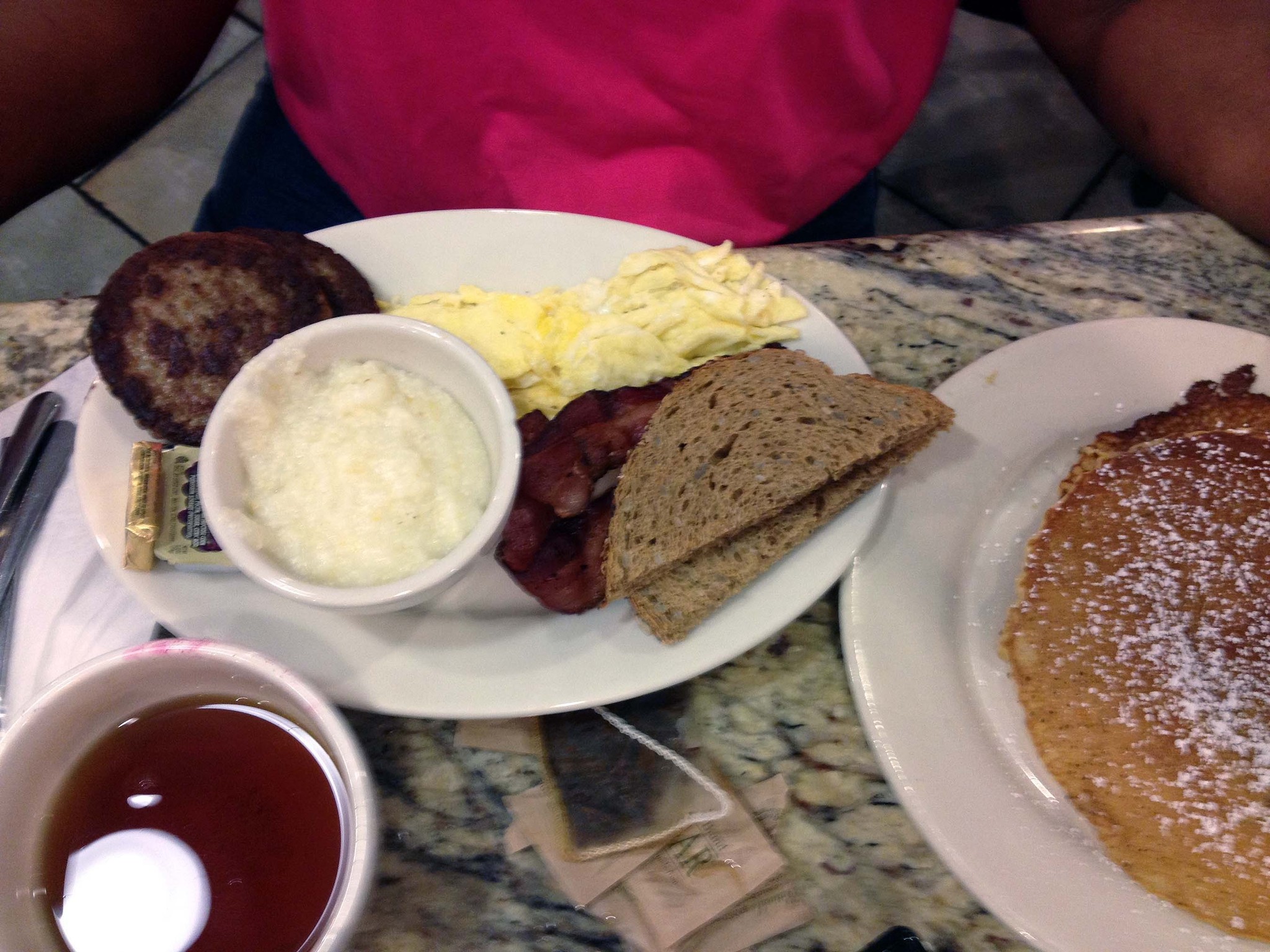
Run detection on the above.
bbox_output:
[839,312,1270,952]
[75,207,891,719]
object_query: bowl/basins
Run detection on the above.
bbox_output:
[198,308,521,618]
[0,637,382,952]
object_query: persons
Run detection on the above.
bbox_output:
[0,0,1270,249]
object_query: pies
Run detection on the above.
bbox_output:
[86,228,377,446]
[996,364,1270,943]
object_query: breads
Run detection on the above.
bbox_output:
[600,347,956,643]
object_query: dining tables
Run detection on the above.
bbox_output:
[1,212,1270,952]
